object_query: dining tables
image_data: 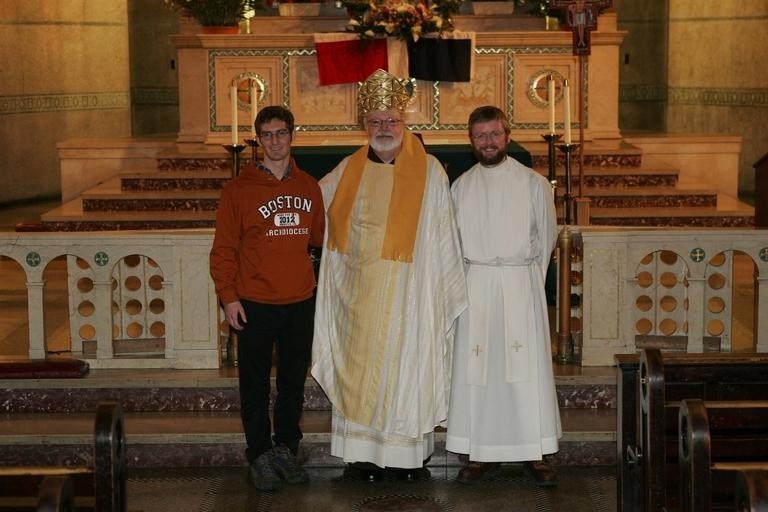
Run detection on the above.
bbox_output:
[163,0,255,34]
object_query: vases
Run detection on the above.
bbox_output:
[344,0,463,51]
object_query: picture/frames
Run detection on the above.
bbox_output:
[168,17,627,143]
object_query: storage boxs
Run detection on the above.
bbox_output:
[614,347,768,512]
[0,359,127,511]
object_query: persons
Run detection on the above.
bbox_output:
[208,106,325,489]
[439,105,563,483]
[310,67,468,485]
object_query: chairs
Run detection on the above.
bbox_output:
[348,461,381,483]
[244,448,283,492]
[269,446,312,487]
[521,457,561,488]
[454,456,504,486]
[397,463,432,483]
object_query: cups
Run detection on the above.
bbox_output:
[470,131,506,140]
[366,117,403,127]
[257,128,291,139]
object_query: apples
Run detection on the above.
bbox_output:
[229,78,238,146]
[563,79,572,143]
[250,81,257,139]
[548,74,556,135]
